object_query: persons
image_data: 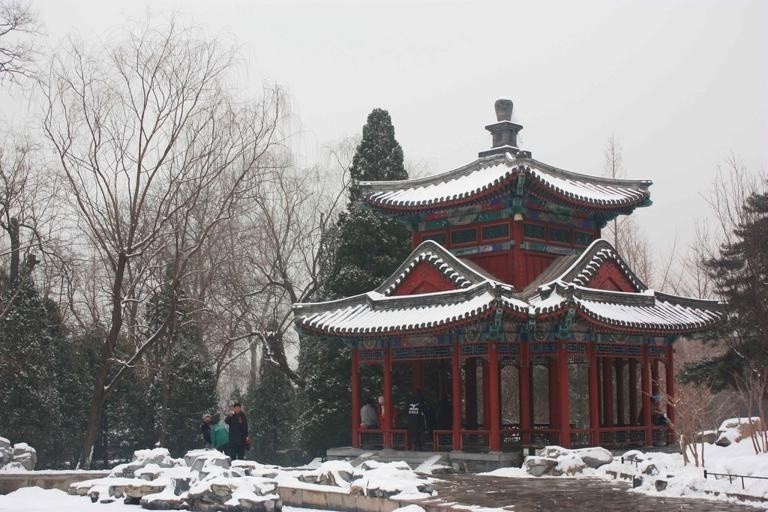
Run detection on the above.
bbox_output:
[207,420,230,457]
[224,403,249,460]
[201,412,214,448]
[360,388,451,451]
[638,399,669,443]
